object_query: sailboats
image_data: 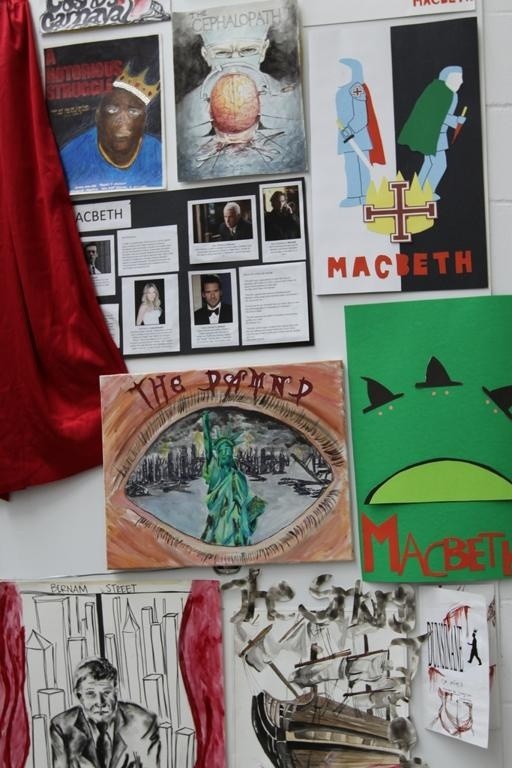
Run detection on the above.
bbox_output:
[239,625,409,767]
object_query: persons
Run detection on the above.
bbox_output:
[201,409,268,548]
[136,281,163,326]
[217,201,253,240]
[56,82,163,187]
[192,274,233,325]
[49,658,162,768]
[176,14,300,172]
[81,240,102,274]
[267,191,299,240]
